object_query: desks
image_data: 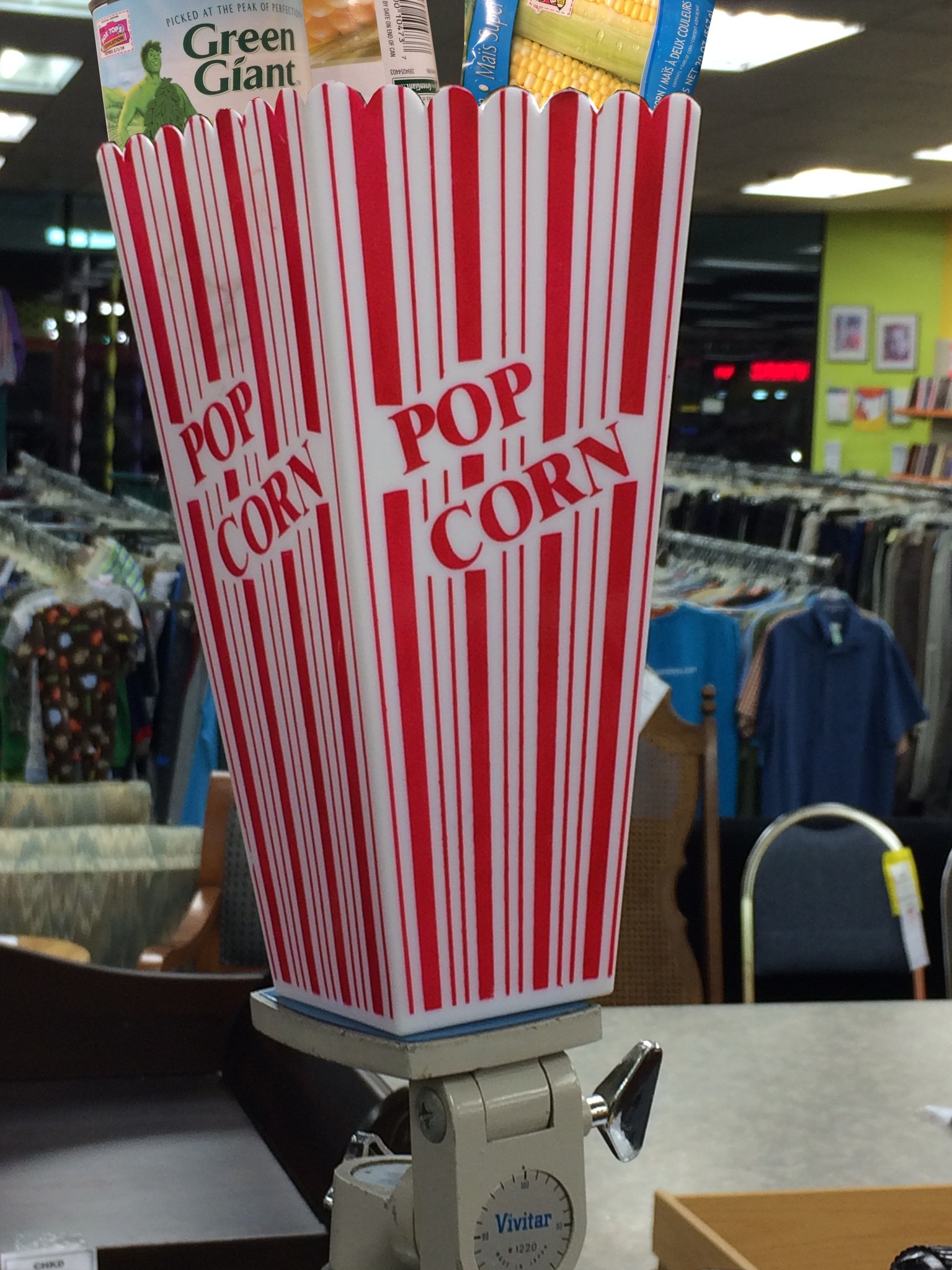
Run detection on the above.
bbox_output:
[351,999,952,1270]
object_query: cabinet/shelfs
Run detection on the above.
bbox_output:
[598,679,727,1006]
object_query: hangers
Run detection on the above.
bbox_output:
[0,454,194,614]
[645,454,952,627]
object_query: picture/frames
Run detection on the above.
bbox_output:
[823,299,923,438]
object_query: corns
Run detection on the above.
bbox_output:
[505,0,660,108]
[303,0,378,52]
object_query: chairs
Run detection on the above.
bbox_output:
[735,796,931,1002]
[129,757,275,974]
